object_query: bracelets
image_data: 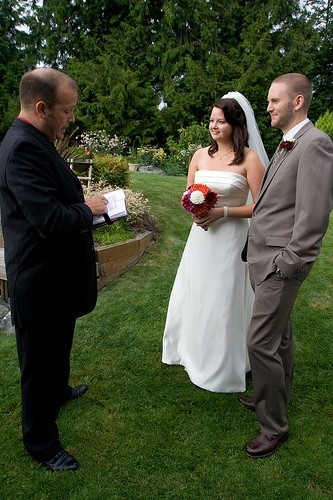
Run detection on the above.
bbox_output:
[224,206,228,218]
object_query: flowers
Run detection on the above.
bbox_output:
[181,183,219,231]
[279,140,294,152]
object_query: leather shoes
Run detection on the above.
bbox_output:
[243,429,289,457]
[42,448,79,471]
[238,395,255,410]
[64,385,88,402]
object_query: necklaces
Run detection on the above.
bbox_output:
[215,149,233,160]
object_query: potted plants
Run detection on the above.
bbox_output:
[126,146,155,172]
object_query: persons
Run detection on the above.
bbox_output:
[0,68,108,472]
[187,98,267,394]
[237,73,333,457]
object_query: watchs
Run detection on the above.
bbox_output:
[276,267,287,279]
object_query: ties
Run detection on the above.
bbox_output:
[266,138,285,179]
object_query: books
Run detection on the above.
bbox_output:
[93,190,128,228]
